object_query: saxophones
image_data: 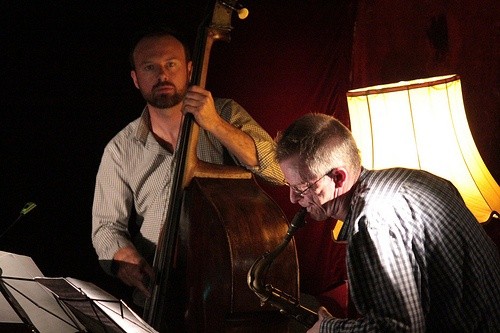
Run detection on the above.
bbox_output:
[247,207,320,330]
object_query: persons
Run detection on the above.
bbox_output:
[90,30,290,321]
[272,112,500,333]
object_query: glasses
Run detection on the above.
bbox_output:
[282,169,335,198]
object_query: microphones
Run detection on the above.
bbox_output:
[272,206,309,242]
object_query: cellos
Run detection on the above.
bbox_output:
[141,0,302,333]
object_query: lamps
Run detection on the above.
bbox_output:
[331,74,499,244]
